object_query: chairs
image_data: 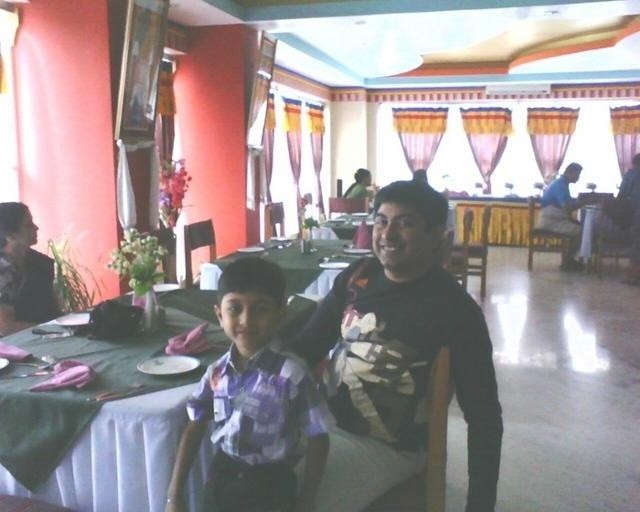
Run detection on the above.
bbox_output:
[118,195,492,303]
[363,342,452,512]
[525,188,638,281]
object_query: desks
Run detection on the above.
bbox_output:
[1,286,323,512]
[448,193,577,253]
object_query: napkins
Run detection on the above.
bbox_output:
[1,342,33,363]
[27,358,98,393]
[166,322,215,356]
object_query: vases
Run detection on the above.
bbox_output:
[131,284,161,332]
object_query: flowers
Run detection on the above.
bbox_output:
[154,155,191,231]
[107,227,168,295]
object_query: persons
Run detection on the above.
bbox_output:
[616,152,640,287]
[342,168,372,200]
[162,258,330,512]
[540,162,595,272]
[286,180,502,512]
[0,200,67,338]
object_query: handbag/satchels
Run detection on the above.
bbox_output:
[87,298,144,340]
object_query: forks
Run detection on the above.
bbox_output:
[87,381,148,405]
[1,357,56,381]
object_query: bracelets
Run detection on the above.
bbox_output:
[165,494,184,507]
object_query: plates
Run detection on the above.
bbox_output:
[134,353,200,375]
[238,233,291,253]
[341,247,374,255]
[51,311,100,328]
[319,261,348,269]
[327,211,374,228]
